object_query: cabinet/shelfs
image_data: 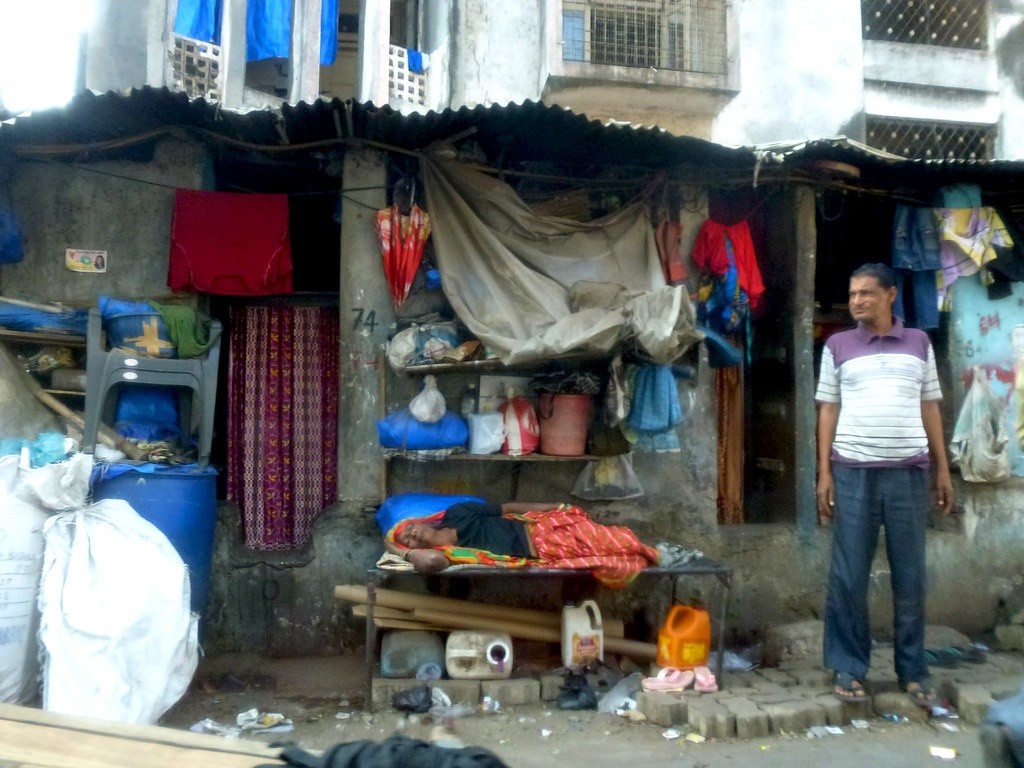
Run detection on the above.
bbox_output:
[386,345,624,462]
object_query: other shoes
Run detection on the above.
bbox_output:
[924,650,957,666]
[952,645,986,662]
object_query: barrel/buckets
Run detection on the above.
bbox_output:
[92,464,220,654]
[539,395,591,456]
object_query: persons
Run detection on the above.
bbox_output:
[383,502,674,570]
[814,264,955,708]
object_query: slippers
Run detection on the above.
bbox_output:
[642,667,694,690]
[693,666,718,692]
[903,680,941,706]
[832,672,866,702]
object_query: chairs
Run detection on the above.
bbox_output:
[83,307,224,469]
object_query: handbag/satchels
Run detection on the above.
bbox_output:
[570,451,644,500]
[949,367,1010,483]
[528,365,604,419]
[467,412,508,454]
[497,387,540,455]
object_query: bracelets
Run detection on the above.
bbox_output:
[404,549,412,560]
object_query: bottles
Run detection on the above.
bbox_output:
[446,632,512,679]
[658,605,711,672]
[382,630,444,680]
[560,601,604,666]
[461,383,478,418]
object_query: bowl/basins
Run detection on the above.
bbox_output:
[107,313,178,356]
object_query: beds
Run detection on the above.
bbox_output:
[361,537,736,714]
